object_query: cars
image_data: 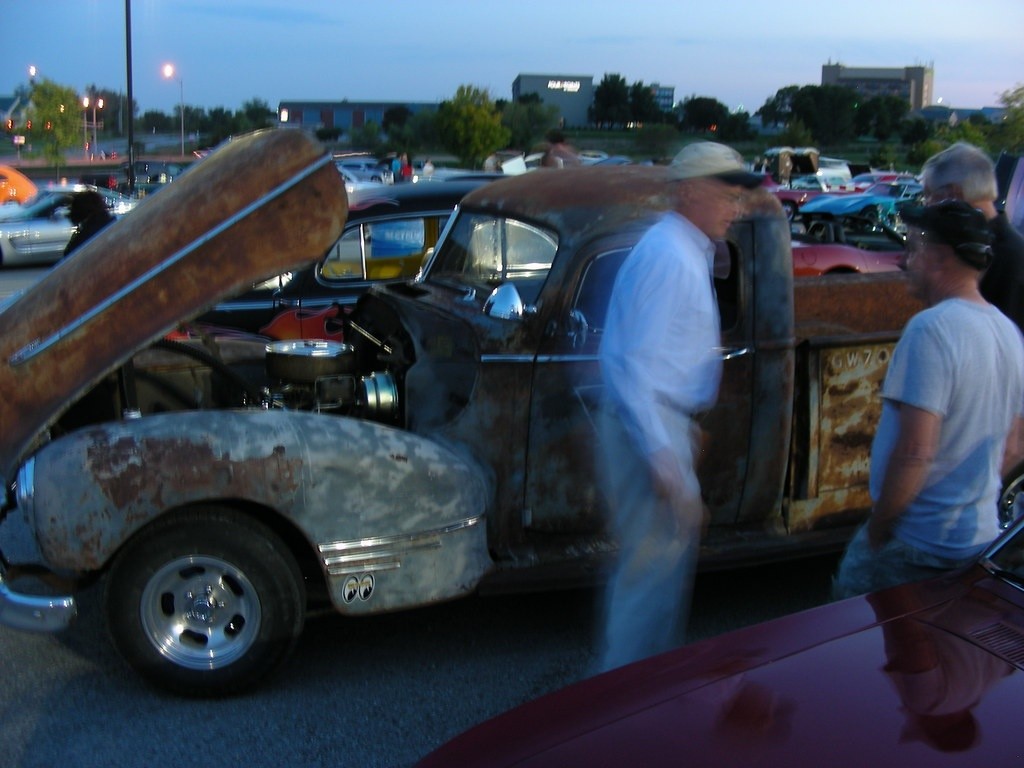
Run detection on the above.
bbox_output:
[0,183,140,275]
[0,166,37,210]
[415,469,1023,768]
[0,129,923,694]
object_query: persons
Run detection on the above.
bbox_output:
[921,142,1024,334]
[423,159,434,174]
[881,618,1016,751]
[392,151,414,184]
[828,196,1024,604]
[541,128,581,170]
[596,140,768,676]
[482,151,502,172]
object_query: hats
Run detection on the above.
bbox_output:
[899,199,993,272]
[666,140,764,191]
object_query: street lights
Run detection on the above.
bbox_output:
[83,97,90,151]
[164,62,185,158]
[93,98,104,154]
